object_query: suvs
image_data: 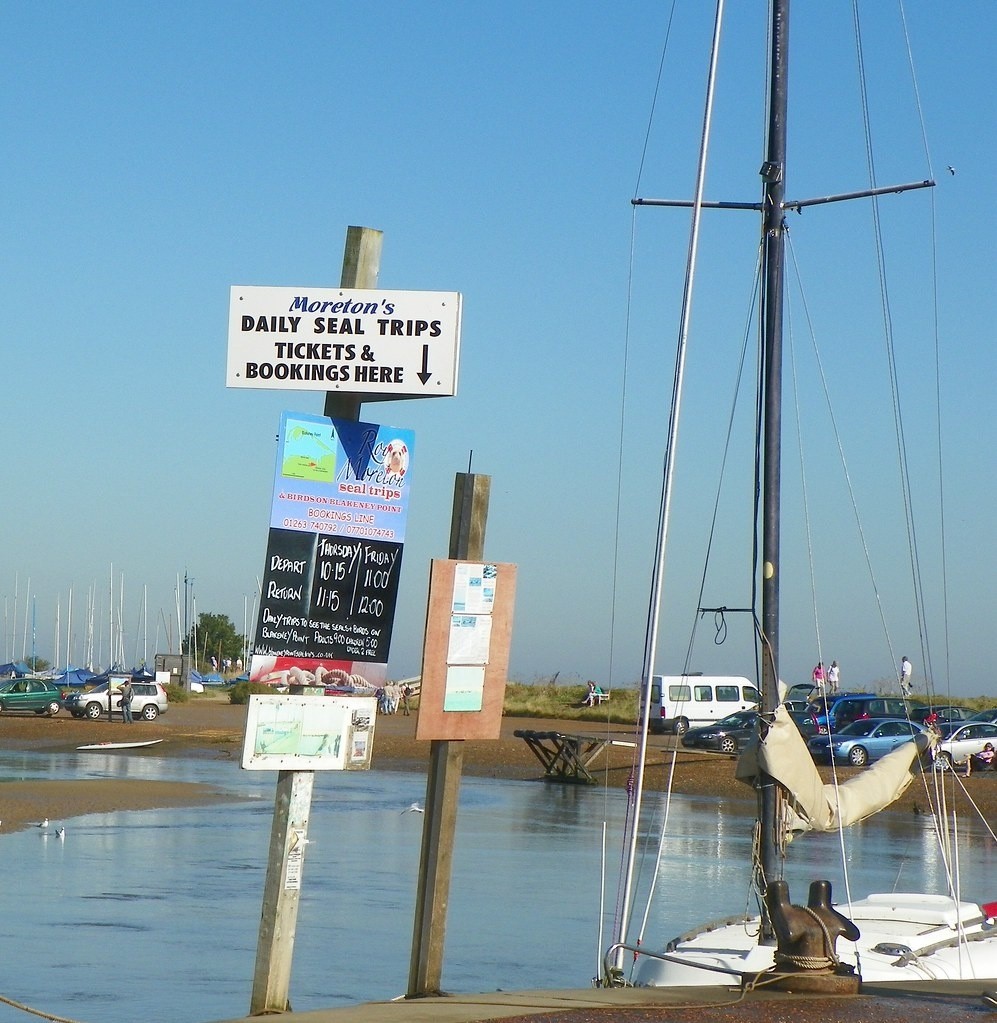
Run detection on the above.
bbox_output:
[0,678,66,718]
[63,677,170,721]
[833,697,929,733]
[910,707,981,728]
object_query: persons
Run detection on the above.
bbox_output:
[221,657,232,673]
[237,657,242,670]
[827,661,840,694]
[901,656,911,697]
[582,680,605,707]
[375,682,412,715]
[958,743,994,777]
[211,657,217,671]
[117,681,135,723]
[813,663,827,696]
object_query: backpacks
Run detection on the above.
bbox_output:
[404,684,410,696]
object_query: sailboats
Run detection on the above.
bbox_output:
[0,567,255,693]
[582,0,997,993]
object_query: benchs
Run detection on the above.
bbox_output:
[594,689,611,706]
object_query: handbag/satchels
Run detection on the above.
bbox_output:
[121,697,129,706]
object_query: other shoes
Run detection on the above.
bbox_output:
[829,690,834,694]
[582,701,587,704]
[590,704,594,707]
[817,694,821,698]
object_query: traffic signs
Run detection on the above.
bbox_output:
[223,284,459,396]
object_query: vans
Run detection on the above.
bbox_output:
[801,693,879,736]
[634,672,762,735]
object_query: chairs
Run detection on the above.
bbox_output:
[971,747,997,777]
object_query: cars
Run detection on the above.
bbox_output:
[808,717,930,766]
[751,683,816,712]
[967,710,997,725]
[929,721,997,773]
[682,710,821,753]
